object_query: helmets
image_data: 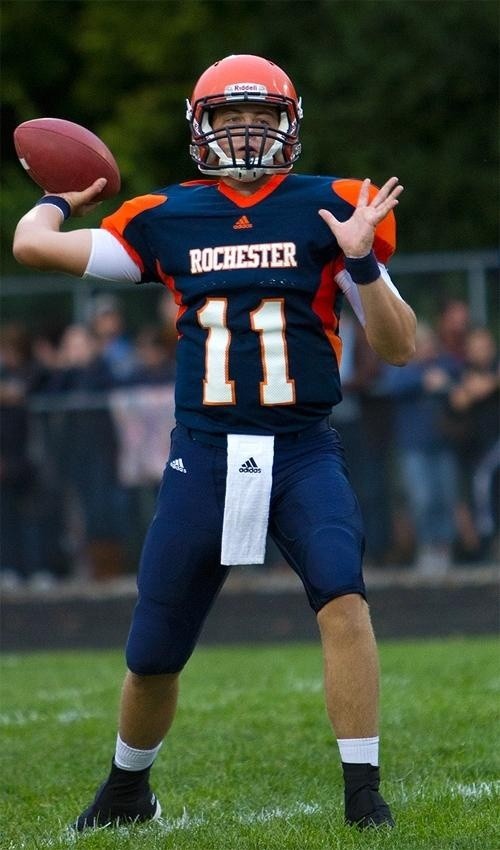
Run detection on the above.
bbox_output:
[186,54,303,169]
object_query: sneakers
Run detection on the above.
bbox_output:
[340,761,396,833]
[66,753,161,833]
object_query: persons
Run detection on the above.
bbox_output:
[13,54,418,832]
[2,287,500,598]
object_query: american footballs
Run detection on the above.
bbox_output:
[15,118,121,202]
